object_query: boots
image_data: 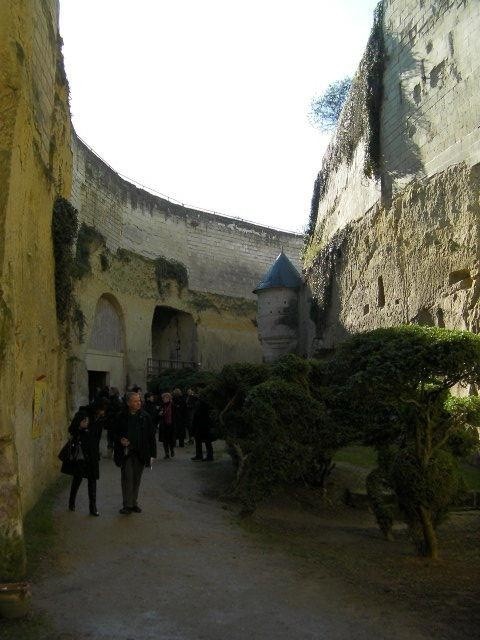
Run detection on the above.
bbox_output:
[89,498,99,516]
[69,496,75,511]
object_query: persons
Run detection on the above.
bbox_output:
[60,384,214,517]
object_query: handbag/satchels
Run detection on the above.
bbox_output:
[58,439,86,464]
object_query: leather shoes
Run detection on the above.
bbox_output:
[119,506,141,513]
